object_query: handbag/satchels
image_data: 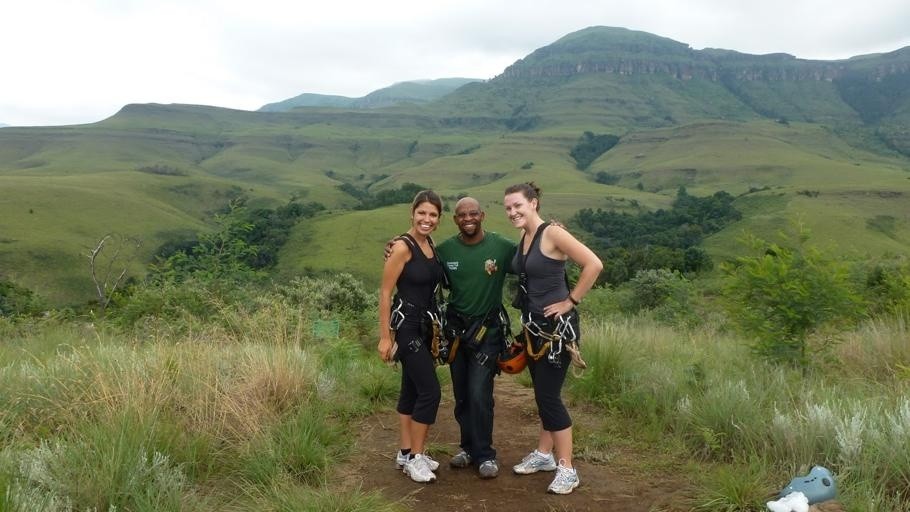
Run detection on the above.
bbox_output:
[447,317,479,338]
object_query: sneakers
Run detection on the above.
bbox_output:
[547,459,579,494]
[449,451,471,467]
[479,460,499,478]
[396,450,440,483]
[513,449,556,474]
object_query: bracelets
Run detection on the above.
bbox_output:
[567,295,582,307]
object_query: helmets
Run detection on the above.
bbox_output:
[498,343,527,374]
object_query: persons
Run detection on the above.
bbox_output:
[380,196,569,479]
[377,189,443,483]
[503,181,604,495]
